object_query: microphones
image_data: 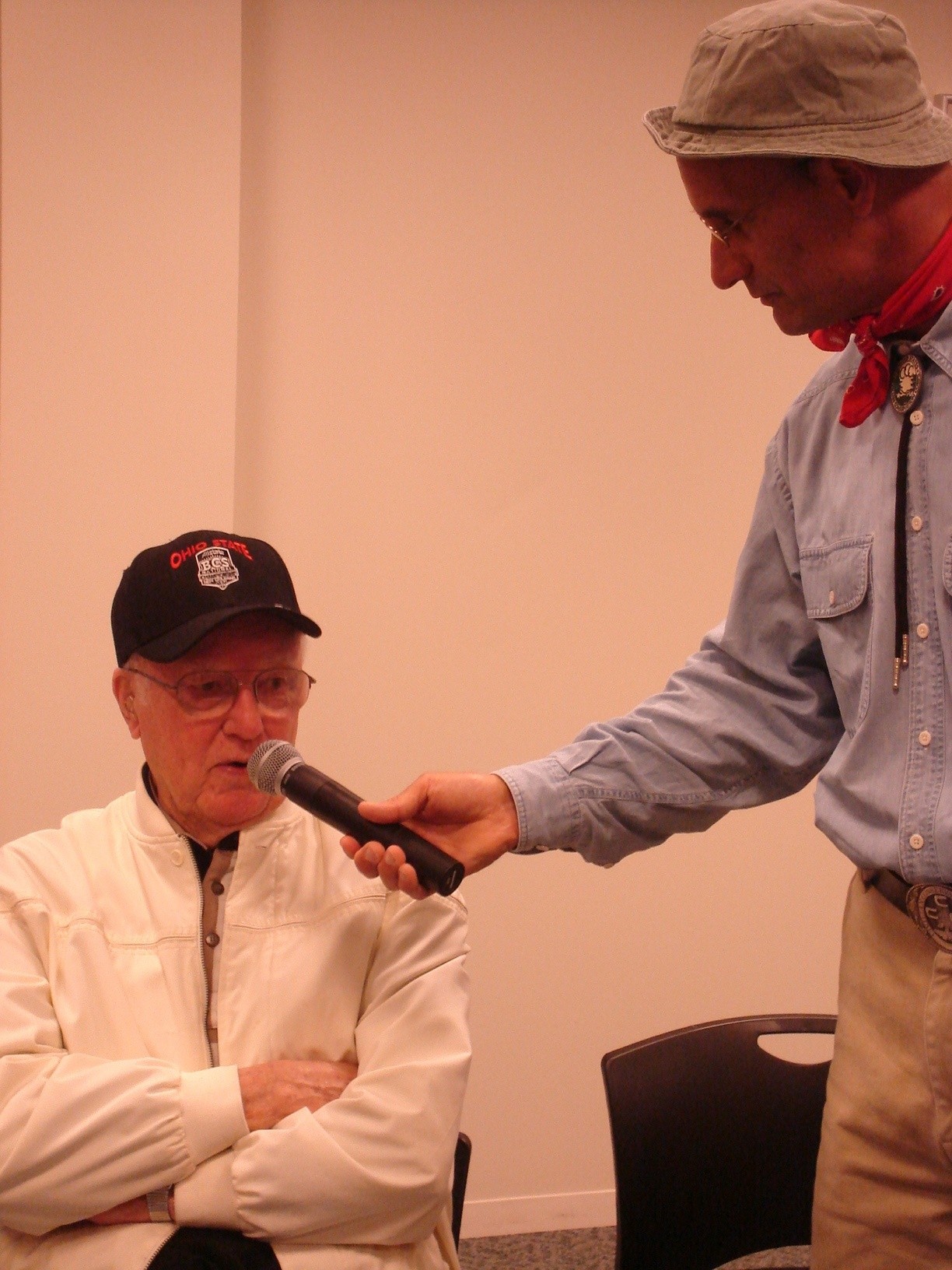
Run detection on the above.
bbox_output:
[246,737,466,896]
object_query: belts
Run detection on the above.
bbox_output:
[858,867,951,956]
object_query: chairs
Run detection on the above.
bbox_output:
[599,1014,836,1270]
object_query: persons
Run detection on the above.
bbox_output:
[340,1,952,1270]
[2,528,470,1270]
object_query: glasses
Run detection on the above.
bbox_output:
[698,154,813,249]
[122,665,316,718]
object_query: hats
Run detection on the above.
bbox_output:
[642,0,952,168]
[110,530,322,669]
